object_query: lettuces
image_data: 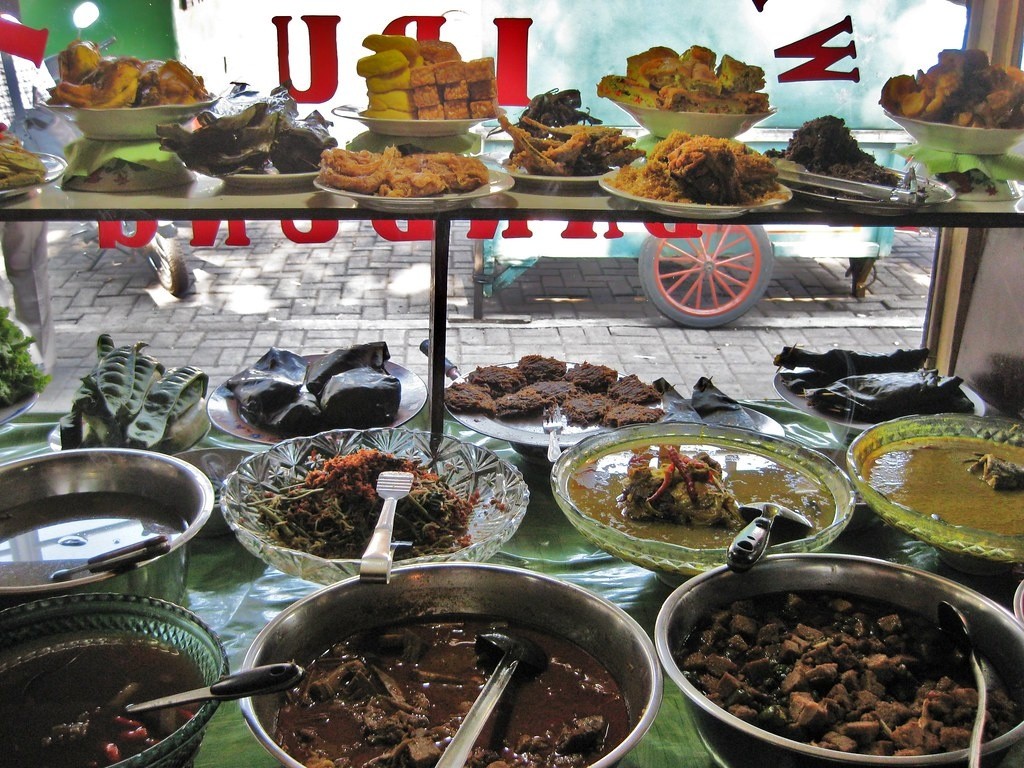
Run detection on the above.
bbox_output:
[0,305,49,404]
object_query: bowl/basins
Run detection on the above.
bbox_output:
[239,561,664,768]
[331,101,507,137]
[170,448,255,537]
[0,591,231,768]
[219,427,530,586]
[445,362,664,466]
[772,364,986,447]
[609,99,777,139]
[845,412,1024,577]
[653,548,1024,767]
[206,352,428,446]
[882,107,1024,154]
[37,95,221,140]
[67,136,194,192]
[1,442,216,618]
[550,421,858,590]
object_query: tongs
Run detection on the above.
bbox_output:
[771,155,919,206]
[360,470,414,586]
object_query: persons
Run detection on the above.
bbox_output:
[0,221,55,376]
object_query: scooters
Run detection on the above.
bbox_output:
[22,1,190,297]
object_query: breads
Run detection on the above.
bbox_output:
[356,33,500,121]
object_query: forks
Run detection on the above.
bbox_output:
[542,403,562,463]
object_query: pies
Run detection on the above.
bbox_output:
[595,45,770,114]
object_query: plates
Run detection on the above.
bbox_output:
[684,398,786,438]
[0,151,68,203]
[219,172,319,189]
[839,168,957,217]
[48,396,209,454]
[0,390,41,427]
[599,169,793,220]
[312,169,516,214]
[472,151,599,191]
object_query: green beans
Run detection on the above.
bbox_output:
[246,456,468,563]
[60,333,210,454]
